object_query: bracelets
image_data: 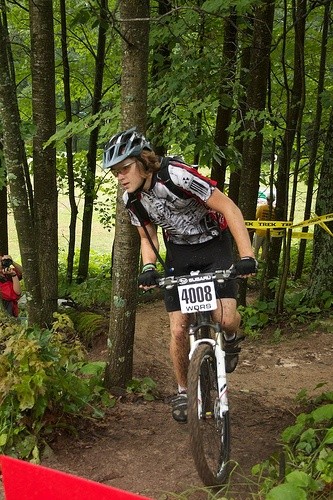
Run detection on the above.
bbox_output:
[142,263,157,272]
[11,274,17,277]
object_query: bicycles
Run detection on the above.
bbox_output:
[142,269,234,494]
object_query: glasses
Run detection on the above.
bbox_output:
[108,161,135,177]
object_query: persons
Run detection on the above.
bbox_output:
[254,193,276,263]
[101,127,258,423]
[0,255,22,318]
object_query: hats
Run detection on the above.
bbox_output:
[268,194,275,200]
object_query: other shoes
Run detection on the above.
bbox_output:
[222,331,239,373]
[170,389,188,424]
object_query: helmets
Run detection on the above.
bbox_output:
[102,131,151,170]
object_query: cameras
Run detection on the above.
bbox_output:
[1,259,12,268]
